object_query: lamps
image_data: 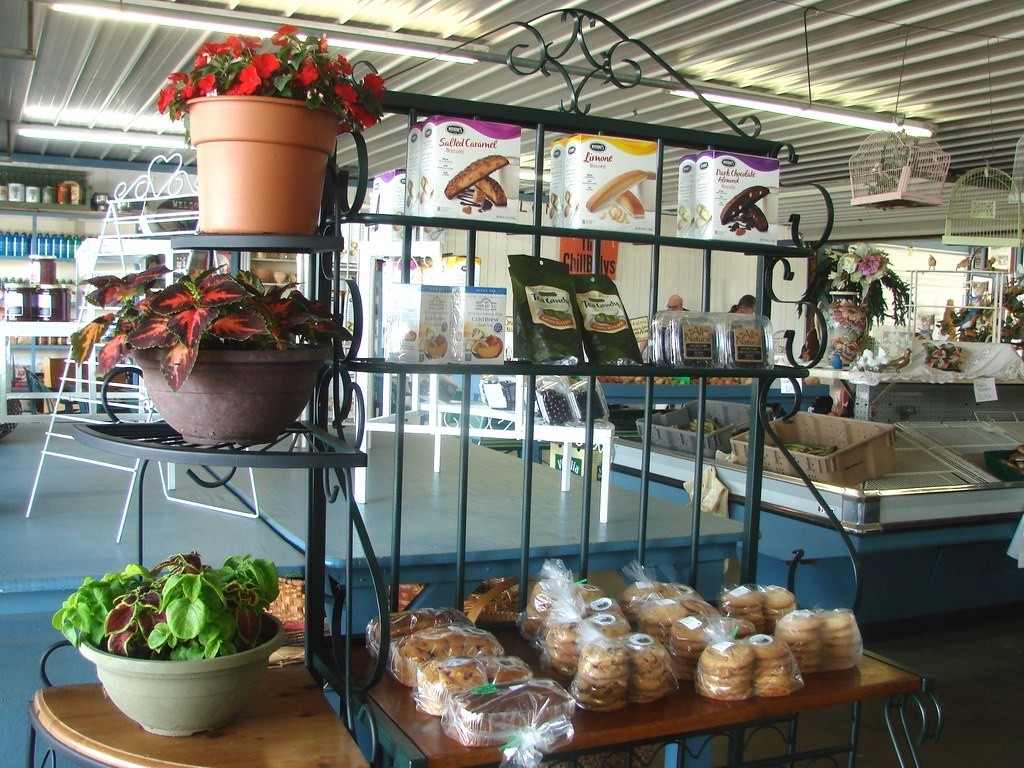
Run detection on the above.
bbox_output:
[656,75,942,142]
[13,123,197,155]
[40,0,485,70]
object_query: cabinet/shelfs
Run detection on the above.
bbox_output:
[0,200,141,265]
[247,250,297,286]
[17,120,378,768]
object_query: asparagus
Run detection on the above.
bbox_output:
[666,412,726,434]
[782,443,840,457]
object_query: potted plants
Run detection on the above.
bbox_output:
[62,267,356,445]
[52,543,294,740]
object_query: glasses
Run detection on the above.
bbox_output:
[666,304,680,309]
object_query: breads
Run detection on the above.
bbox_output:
[720,185,770,232]
[586,169,648,218]
[999,444,1024,473]
[368,606,572,747]
[444,154,509,207]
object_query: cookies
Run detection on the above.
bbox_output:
[521,579,854,712]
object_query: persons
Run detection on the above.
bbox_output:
[640,295,690,353]
[731,294,755,314]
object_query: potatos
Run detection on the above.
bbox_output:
[597,375,753,384]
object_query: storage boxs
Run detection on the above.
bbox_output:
[0,163,93,211]
[12,115,895,486]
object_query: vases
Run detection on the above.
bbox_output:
[187,96,335,232]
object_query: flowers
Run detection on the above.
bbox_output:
[153,26,387,135]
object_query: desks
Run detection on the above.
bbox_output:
[314,633,945,768]
